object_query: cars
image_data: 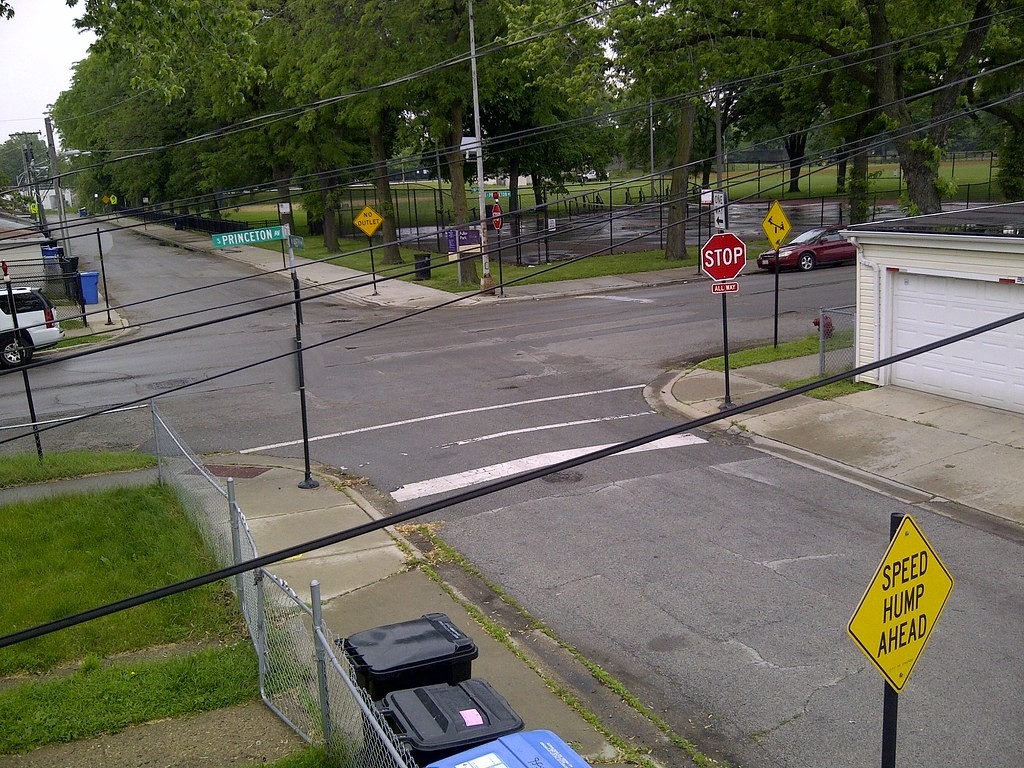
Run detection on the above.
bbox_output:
[757,224,856,271]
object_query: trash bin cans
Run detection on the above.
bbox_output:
[415,254,432,280]
[80,271,99,302]
[422,730,594,767]
[344,615,480,768]
[374,678,524,768]
[80,209,87,217]
[61,257,79,301]
[42,246,63,266]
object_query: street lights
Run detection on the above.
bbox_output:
[30,149,80,256]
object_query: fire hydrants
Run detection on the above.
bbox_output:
[812,315,835,339]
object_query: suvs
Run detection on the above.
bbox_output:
[0,287,65,368]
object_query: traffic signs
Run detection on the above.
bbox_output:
[713,191,729,230]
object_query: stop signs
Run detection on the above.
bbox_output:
[492,204,503,229]
[701,233,746,282]
